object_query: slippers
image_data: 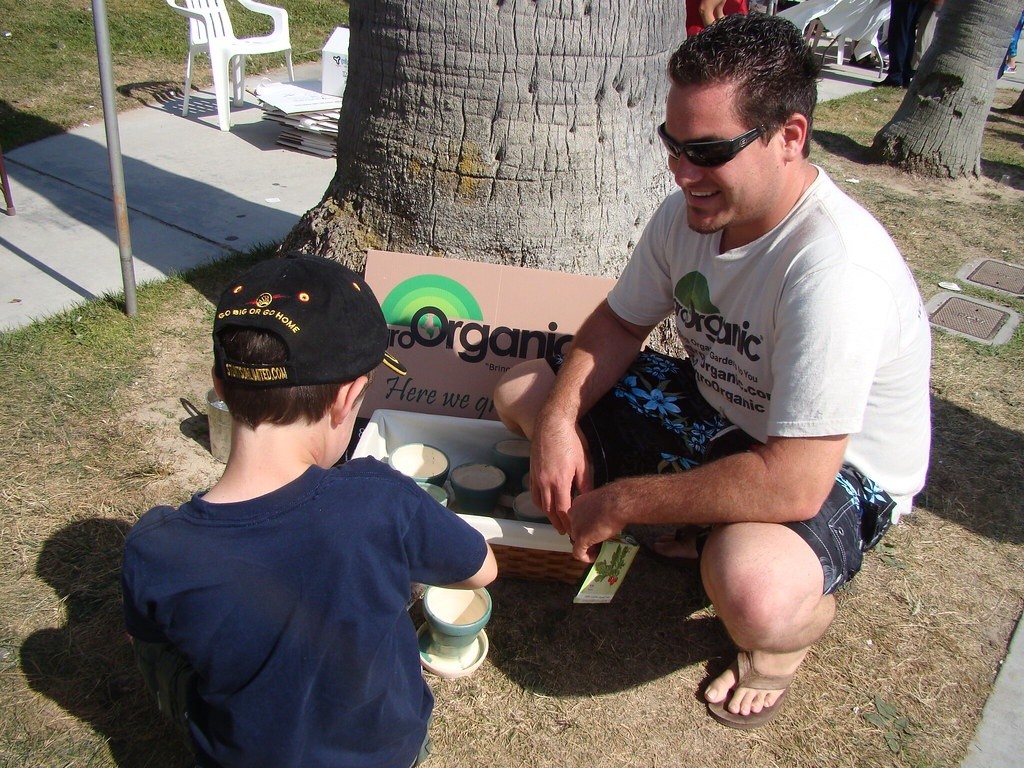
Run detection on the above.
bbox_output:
[706,648,797,729]
[647,531,711,570]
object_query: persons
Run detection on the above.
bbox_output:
[494,13,931,730]
[686,0,748,38]
[910,0,944,77]
[1003,11,1024,74]
[119,249,498,768]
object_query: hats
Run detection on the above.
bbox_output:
[213,251,408,391]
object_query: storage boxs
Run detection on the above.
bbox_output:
[350,413,591,583]
[321,26,350,97]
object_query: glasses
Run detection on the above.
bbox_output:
[658,122,777,169]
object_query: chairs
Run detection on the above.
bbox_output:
[808,19,856,65]
[167,0,294,132]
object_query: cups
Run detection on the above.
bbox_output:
[387,443,451,487]
[492,439,530,514]
[513,490,551,524]
[449,463,506,517]
[424,584,492,657]
[416,481,448,508]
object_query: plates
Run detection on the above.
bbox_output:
[416,622,490,678]
